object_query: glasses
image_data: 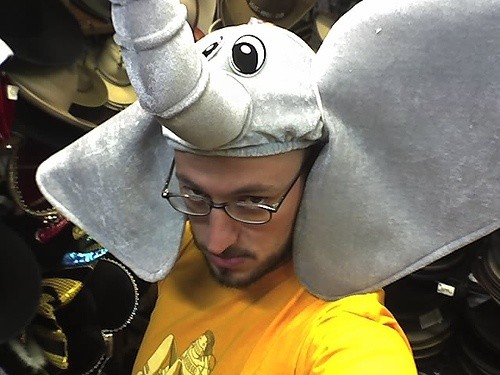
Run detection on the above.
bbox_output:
[161,151,305,225]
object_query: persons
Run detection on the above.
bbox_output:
[131,17,421,375]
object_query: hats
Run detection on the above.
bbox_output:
[84,37,138,112]
[72,64,107,107]
[315,0,357,42]
[0,133,139,375]
[179,0,217,38]
[0,52,98,131]
[385,228,499,375]
[219,0,316,30]
